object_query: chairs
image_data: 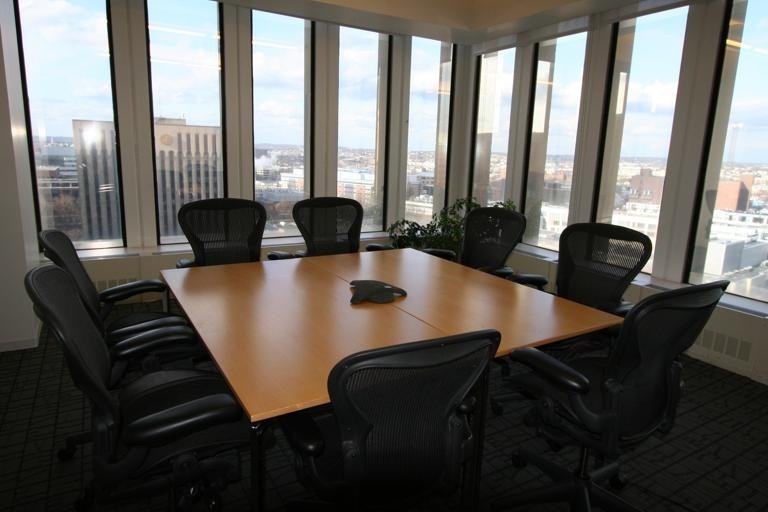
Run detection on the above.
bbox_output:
[37,227,197,365]
[423,206,527,276]
[177,196,268,267]
[503,280,730,509]
[270,197,364,261]
[304,328,503,509]
[490,221,651,439]
[23,264,250,512]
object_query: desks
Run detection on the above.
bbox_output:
[303,245,625,358]
[159,256,452,426]
